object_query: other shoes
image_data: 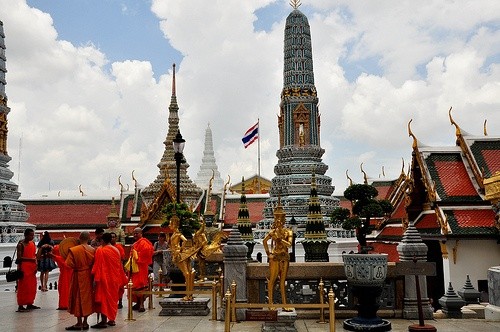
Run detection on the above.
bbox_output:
[66,323,83,330]
[83,324,89,330]
[17,307,29,311]
[117,303,122,308]
[108,321,115,326]
[91,322,107,328]
[139,308,145,312]
[27,305,40,309]
[132,303,139,310]
[41,287,48,291]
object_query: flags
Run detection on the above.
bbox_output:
[242,122,259,149]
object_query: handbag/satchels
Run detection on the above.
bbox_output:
[6,269,22,282]
[153,253,163,263]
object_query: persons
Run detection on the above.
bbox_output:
[169,215,229,301]
[124,227,155,312]
[262,194,293,305]
[105,197,126,246]
[42,229,129,330]
[152,234,169,295]
[15,228,42,311]
[37,231,57,291]
